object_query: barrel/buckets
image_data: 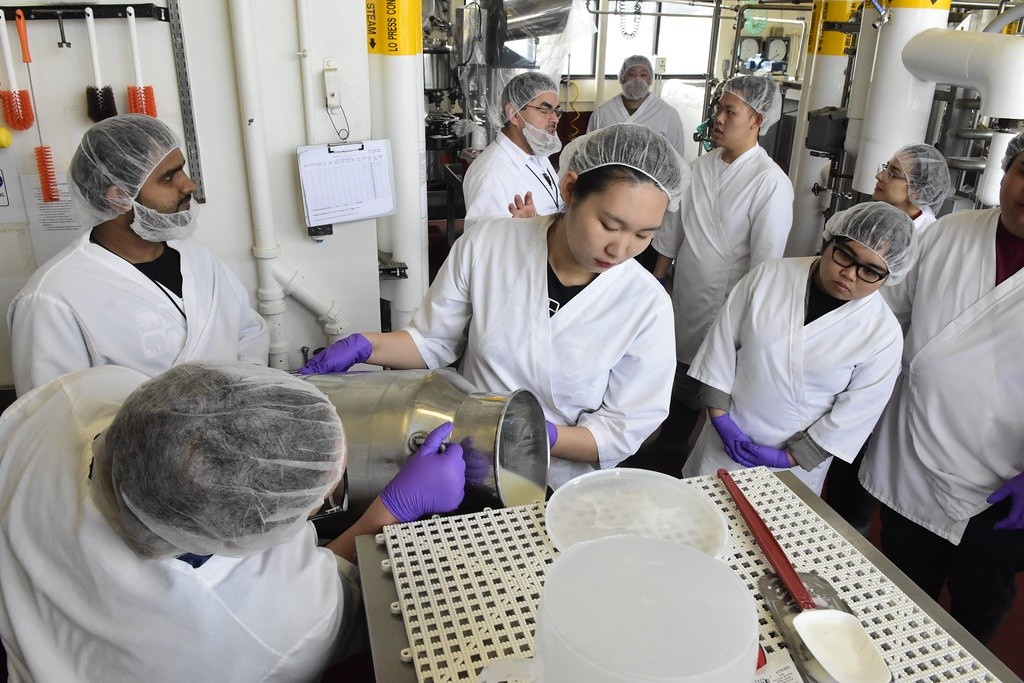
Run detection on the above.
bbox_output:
[286,367,550,510]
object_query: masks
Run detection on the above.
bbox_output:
[129,201,199,242]
[622,76,649,101]
[517,112,562,159]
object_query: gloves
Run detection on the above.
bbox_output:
[380,423,465,523]
[988,472,1024,532]
[457,435,488,485]
[711,413,754,470]
[303,333,373,382]
[724,437,791,469]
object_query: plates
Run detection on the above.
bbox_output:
[546,467,728,562]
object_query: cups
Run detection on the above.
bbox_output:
[471,126,488,150]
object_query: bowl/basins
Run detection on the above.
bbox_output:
[535,534,758,682]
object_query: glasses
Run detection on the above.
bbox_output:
[525,104,563,119]
[877,163,926,184]
[309,464,350,522]
[832,237,890,285]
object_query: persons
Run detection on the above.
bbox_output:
[586,54,683,160]
[823,131,1024,645]
[5,112,268,401]
[296,124,692,491]
[0,360,466,683]
[682,201,918,477]
[462,75,565,233]
[625,76,794,467]
[823,144,951,537]
